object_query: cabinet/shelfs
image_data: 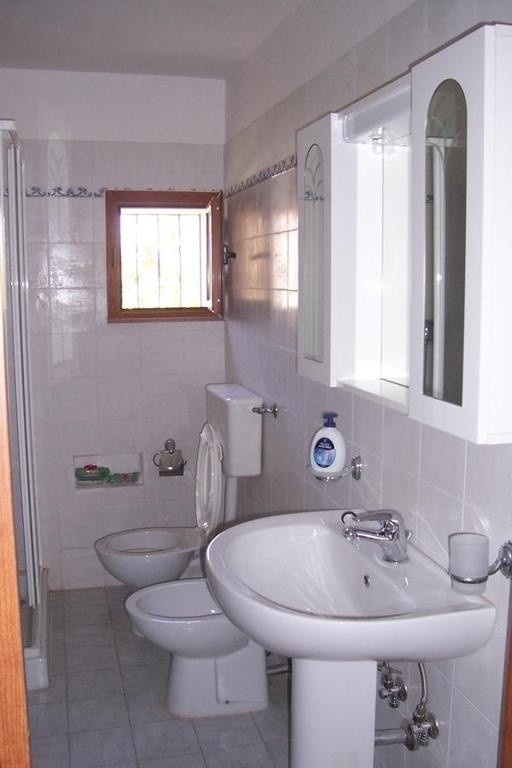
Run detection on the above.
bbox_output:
[409,21,511,449]
[294,114,339,391]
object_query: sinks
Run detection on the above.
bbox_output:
[124,578,269,718]
[205,508,495,660]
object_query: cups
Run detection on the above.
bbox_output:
[450,532,489,596]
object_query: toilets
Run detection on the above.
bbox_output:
[95,383,263,639]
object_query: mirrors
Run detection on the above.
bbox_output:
[339,111,409,389]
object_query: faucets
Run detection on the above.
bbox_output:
[343,509,408,563]
